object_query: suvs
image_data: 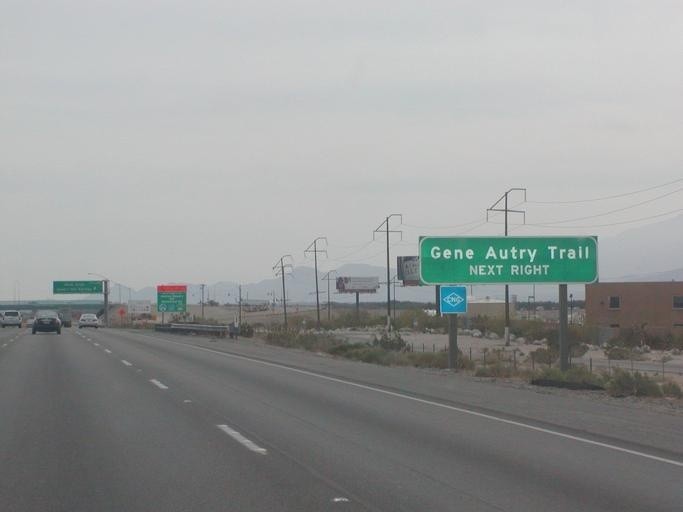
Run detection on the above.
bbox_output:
[1,310,99,334]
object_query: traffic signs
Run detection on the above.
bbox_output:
[53,281,104,293]
[439,287,466,314]
[419,236,598,283]
[158,285,186,312]
